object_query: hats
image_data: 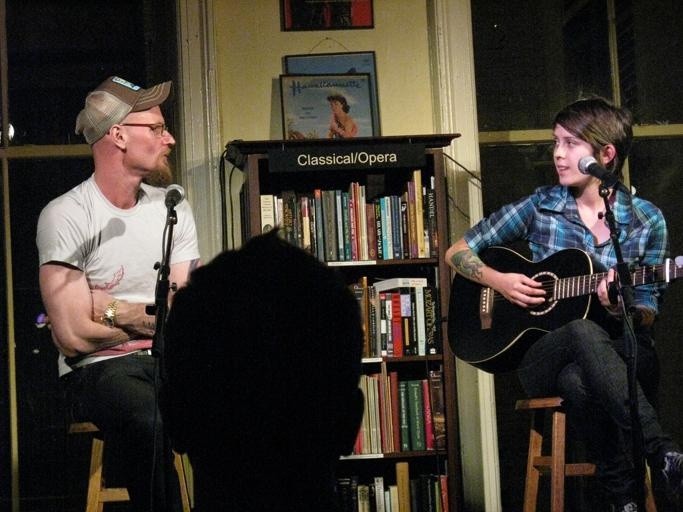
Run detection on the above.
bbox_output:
[76,76,172,145]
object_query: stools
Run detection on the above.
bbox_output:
[516,397,657,512]
[69,419,202,512]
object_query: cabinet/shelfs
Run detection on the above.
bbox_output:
[225,133,463,512]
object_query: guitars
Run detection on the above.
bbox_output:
[448,245,683,375]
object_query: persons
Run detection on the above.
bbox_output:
[154,222,365,511]
[442,90,682,511]
[33,74,205,512]
[323,93,357,138]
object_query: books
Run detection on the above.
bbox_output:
[260,169,439,262]
[336,461,448,511]
[349,277,440,359]
[354,362,444,455]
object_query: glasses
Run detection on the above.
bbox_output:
[121,123,166,137]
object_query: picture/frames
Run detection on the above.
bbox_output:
[277,0,381,137]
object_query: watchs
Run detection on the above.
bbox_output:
[100,298,119,331]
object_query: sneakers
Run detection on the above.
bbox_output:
[660,452,683,482]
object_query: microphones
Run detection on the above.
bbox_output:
[163,183,187,208]
[578,155,633,196]
